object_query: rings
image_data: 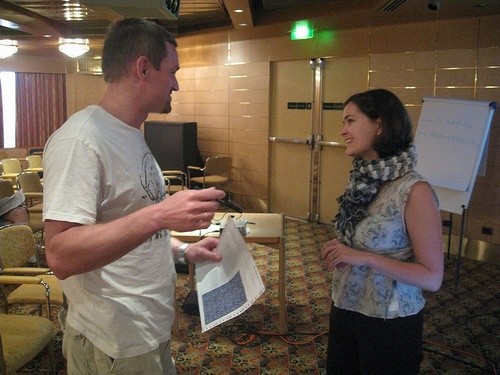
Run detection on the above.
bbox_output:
[203,211,207,219]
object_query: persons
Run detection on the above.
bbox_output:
[323,89,446,375]
[43,16,224,375]
[0,206,37,264]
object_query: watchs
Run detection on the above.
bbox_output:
[177,242,191,265]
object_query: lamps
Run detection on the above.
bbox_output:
[59,42,90,60]
[290,20,314,40]
[0,44,18,60]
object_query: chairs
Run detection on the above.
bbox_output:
[17,214,43,241]
[1,158,22,190]
[162,170,187,195]
[18,173,43,221]
[0,224,62,317]
[188,156,232,202]
[27,155,44,173]
[0,276,58,375]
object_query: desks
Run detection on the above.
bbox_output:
[169,212,288,335]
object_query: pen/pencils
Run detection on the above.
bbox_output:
[215,196,244,213]
